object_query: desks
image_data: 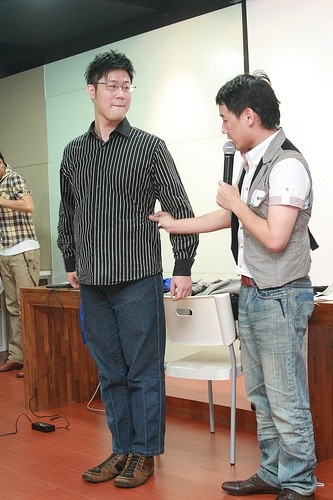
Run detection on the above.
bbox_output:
[18,282,332,463]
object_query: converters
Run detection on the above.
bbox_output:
[31,421,55,432]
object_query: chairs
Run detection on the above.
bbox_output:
[162,293,243,465]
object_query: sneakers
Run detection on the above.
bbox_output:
[82,453,128,483]
[114,453,154,487]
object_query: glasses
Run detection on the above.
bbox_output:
[88,81,136,93]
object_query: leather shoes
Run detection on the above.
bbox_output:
[0,358,23,372]
[275,488,315,500]
[16,367,24,377]
[222,473,281,495]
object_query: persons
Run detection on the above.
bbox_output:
[146,68,318,500]
[55,50,199,490]
[0,152,42,379]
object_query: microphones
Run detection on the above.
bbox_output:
[222,141,237,186]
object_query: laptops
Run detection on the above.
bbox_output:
[45,282,74,288]
